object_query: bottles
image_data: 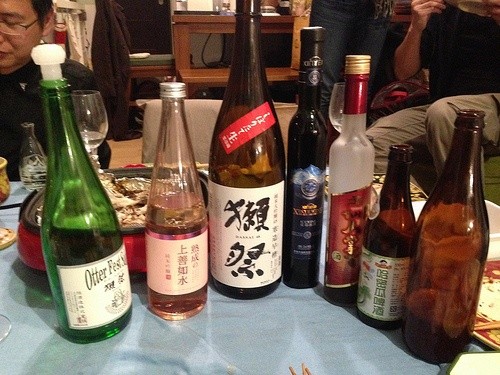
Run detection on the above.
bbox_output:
[146,83,209,321]
[357,144,417,330]
[284,27,327,291]
[325,55,375,289]
[30,43,132,344]
[16,120,50,192]
[402,109,490,363]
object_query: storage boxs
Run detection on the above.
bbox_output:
[412,199,499,261]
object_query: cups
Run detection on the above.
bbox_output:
[0,155,12,207]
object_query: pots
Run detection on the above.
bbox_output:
[210,0,286,298]
[16,166,209,272]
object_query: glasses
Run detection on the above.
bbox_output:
[0,18,41,36]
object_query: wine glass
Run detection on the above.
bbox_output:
[327,80,354,139]
[66,86,110,173]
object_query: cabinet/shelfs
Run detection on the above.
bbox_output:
[171,14,417,99]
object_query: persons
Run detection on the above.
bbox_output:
[365,0,500,199]
[0,0,112,182]
[309,1,395,123]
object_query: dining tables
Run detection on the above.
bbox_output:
[0,181,452,375]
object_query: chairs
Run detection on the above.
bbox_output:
[124,54,176,100]
[412,155,500,204]
[142,99,223,163]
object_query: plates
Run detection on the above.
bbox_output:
[443,348,500,375]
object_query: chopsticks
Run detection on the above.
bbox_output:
[473,318,500,339]
[289,362,308,375]
